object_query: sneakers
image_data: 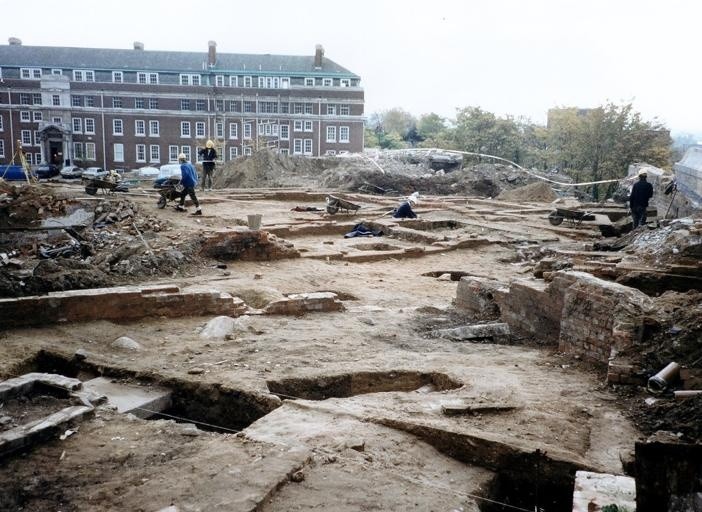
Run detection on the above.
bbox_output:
[192,206,201,214]
[178,205,184,209]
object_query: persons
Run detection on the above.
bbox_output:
[198,139,217,191]
[629,168,653,230]
[395,196,418,218]
[174,153,202,215]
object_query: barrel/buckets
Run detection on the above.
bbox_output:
[245,213,264,230]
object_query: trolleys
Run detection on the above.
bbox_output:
[544,206,592,227]
[154,177,187,210]
[80,177,120,197]
[323,194,362,218]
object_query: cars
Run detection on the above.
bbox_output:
[59,165,82,179]
[80,167,108,180]
[138,167,159,179]
[34,164,61,179]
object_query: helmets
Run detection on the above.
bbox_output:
[206,140,213,148]
[408,195,417,205]
[178,153,186,158]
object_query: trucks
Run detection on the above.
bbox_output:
[159,164,182,178]
